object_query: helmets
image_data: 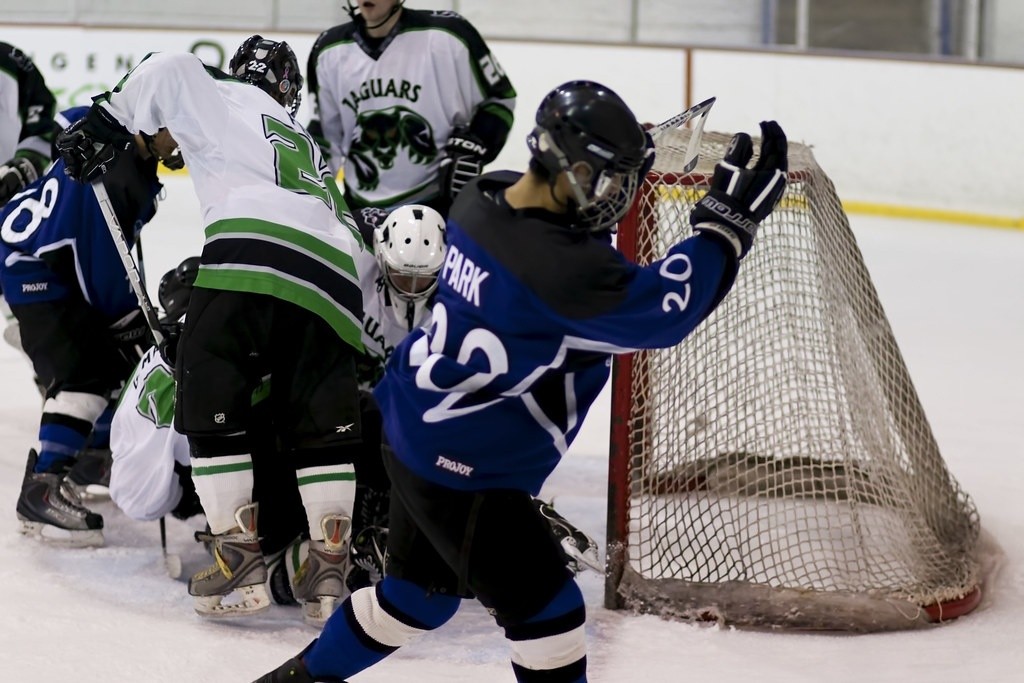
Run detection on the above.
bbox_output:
[230,34,304,119]
[525,80,647,228]
[373,204,449,302]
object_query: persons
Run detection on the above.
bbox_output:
[107,202,610,591]
[306,0,516,245]
[0,97,184,547]
[253,81,789,683]
[56,35,364,625]
[0,40,58,353]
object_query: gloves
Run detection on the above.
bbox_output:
[437,128,487,212]
[107,309,155,366]
[57,102,133,185]
[688,121,788,259]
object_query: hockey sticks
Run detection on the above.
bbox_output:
[135,234,184,581]
[642,94,718,176]
[69,128,168,346]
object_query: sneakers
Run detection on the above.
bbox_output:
[355,528,390,584]
[293,512,352,626]
[189,502,272,614]
[65,448,113,497]
[536,497,605,574]
[15,447,105,549]
